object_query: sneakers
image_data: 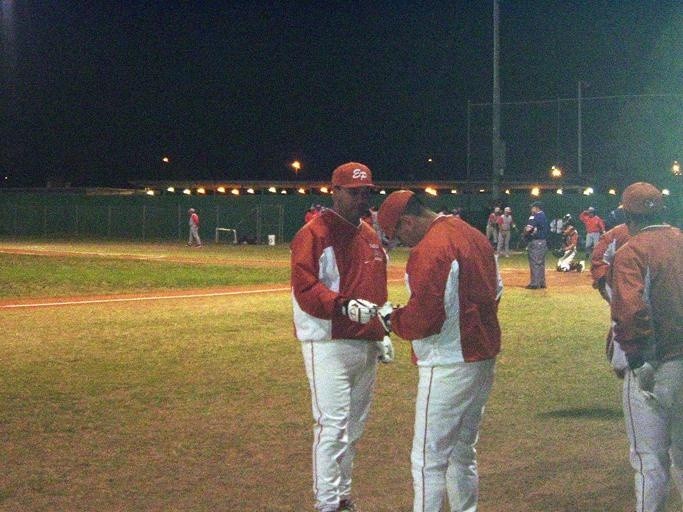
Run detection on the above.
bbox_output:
[525,285,545,289]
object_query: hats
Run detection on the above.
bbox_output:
[530,201,544,207]
[377,189,414,241]
[584,206,595,213]
[622,183,662,216]
[494,207,511,213]
[331,163,377,190]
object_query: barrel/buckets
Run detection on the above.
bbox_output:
[268,235,275,246]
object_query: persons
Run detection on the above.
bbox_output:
[184,208,201,248]
[302,201,397,254]
[603,179,683,512]
[289,160,396,512]
[592,220,630,379]
[437,194,625,290]
[375,189,504,512]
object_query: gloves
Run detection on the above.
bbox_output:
[341,297,376,324]
[377,336,394,364]
[633,364,656,402]
[378,301,393,331]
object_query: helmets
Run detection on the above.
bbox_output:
[563,213,575,227]
